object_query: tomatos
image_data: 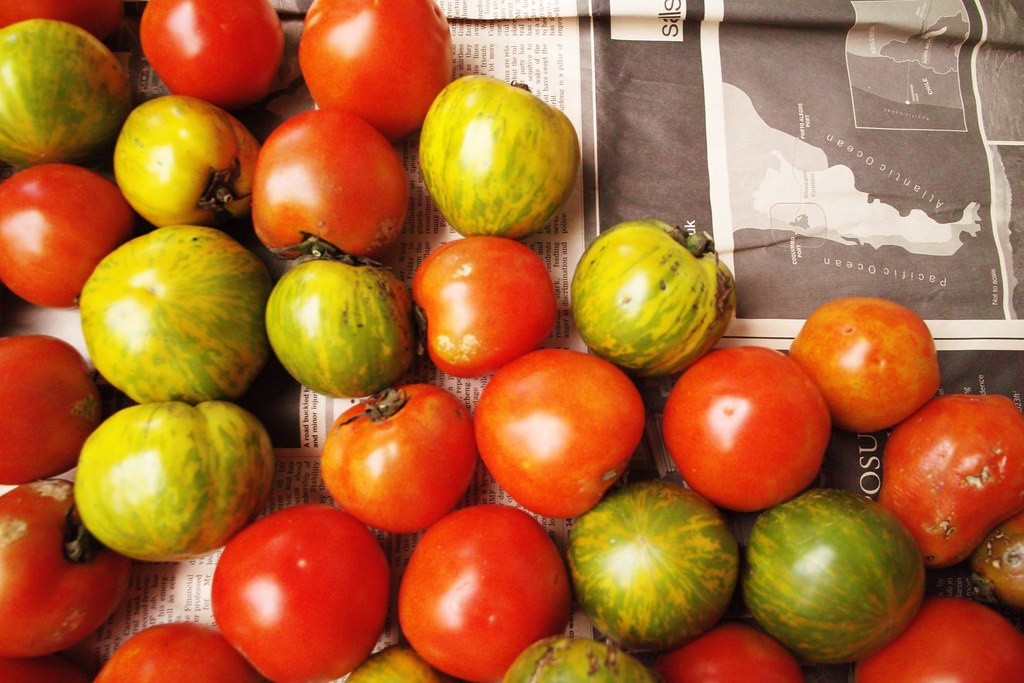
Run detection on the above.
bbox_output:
[0,0,1024,683]
